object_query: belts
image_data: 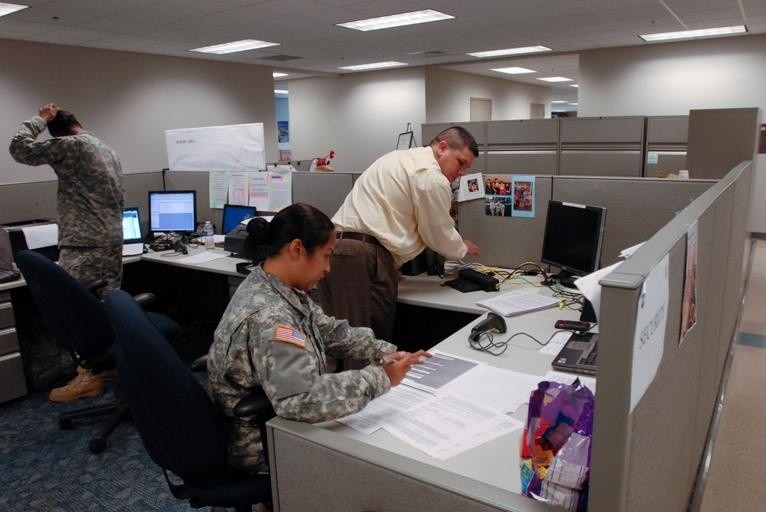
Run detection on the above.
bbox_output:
[336,232,380,245]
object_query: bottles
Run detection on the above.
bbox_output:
[204,221,215,249]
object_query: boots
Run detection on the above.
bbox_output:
[49,366,119,402]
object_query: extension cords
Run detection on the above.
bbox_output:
[459,269,497,290]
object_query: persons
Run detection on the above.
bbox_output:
[9,102,126,401]
[486,178,509,195]
[471,182,478,192]
[317,126,480,346]
[516,187,531,210]
[490,199,505,216]
[208,203,432,478]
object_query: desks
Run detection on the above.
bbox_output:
[0,243,597,512]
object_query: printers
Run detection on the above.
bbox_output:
[2,217,60,272]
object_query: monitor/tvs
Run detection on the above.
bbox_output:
[222,206,256,235]
[540,201,607,295]
[122,207,143,243]
[147,191,197,232]
